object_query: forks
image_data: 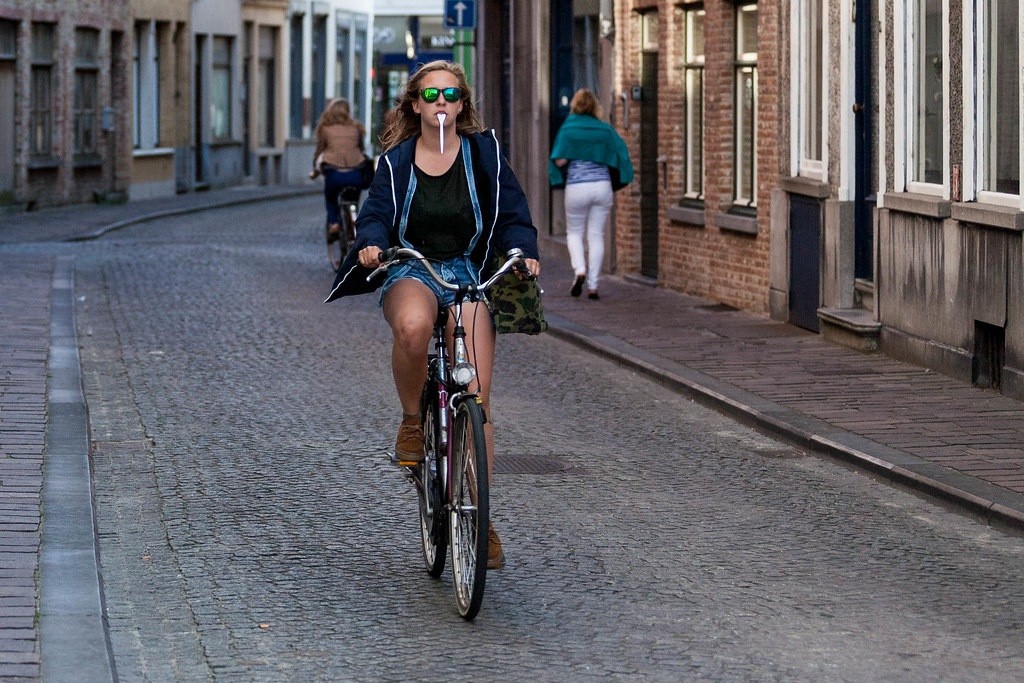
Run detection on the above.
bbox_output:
[437,113,446,154]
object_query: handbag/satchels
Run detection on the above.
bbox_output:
[491,252,549,336]
[362,153,374,191]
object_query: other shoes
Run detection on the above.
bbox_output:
[570,270,587,297]
[588,287,600,299]
[395,414,426,461]
[326,222,341,246]
[472,516,504,568]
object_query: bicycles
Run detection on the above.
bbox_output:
[364,246,545,620]
[308,170,359,272]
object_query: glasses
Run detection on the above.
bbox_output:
[417,87,463,103]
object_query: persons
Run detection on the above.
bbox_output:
[324,62,548,570]
[309,97,375,245]
[546,87,634,300]
